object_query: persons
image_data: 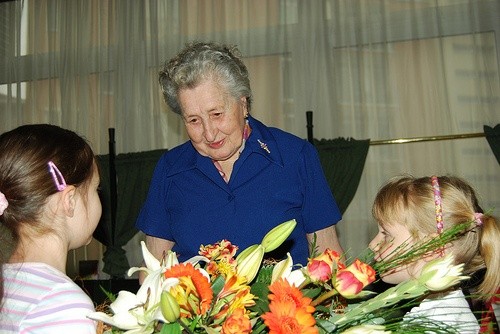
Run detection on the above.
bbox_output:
[367,173,500,334]
[137,42,346,288]
[0,124,102,334]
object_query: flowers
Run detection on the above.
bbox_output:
[83,210,495,334]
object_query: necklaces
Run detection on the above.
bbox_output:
[212,120,249,183]
